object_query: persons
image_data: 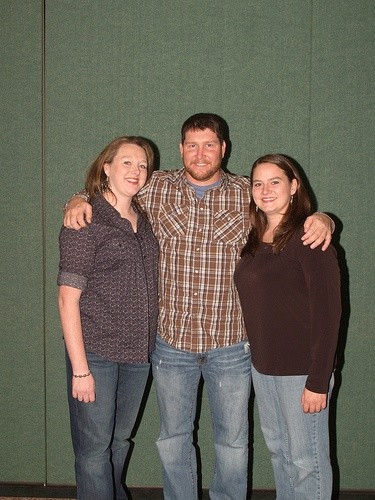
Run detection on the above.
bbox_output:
[56,135,163,500]
[231,152,346,500]
[60,111,337,500]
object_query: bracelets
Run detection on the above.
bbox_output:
[72,370,91,378]
